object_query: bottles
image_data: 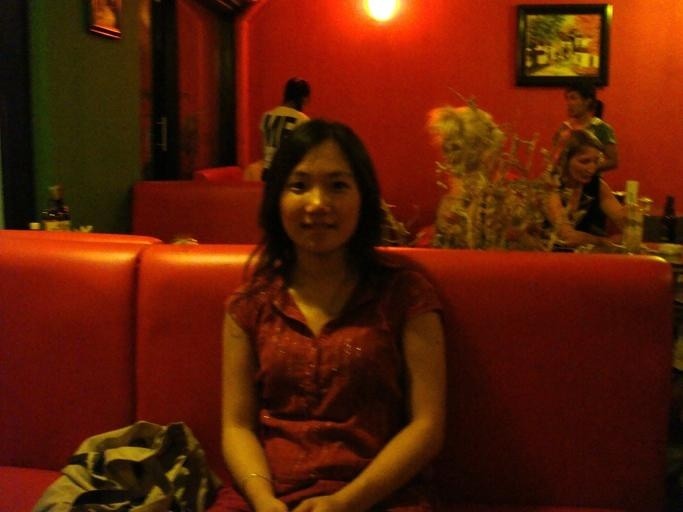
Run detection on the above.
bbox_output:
[657,195,678,244]
[41,182,72,232]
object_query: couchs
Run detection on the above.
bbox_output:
[0,167,672,511]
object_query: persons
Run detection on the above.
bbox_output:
[548,82,618,173]
[532,129,632,248]
[146,134,202,180]
[220,119,450,511]
[259,77,311,181]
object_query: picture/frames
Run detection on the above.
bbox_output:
[86,1,124,42]
[514,3,612,88]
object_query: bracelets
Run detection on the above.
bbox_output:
[239,473,272,490]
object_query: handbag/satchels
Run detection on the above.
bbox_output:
[31,421,223,512]
